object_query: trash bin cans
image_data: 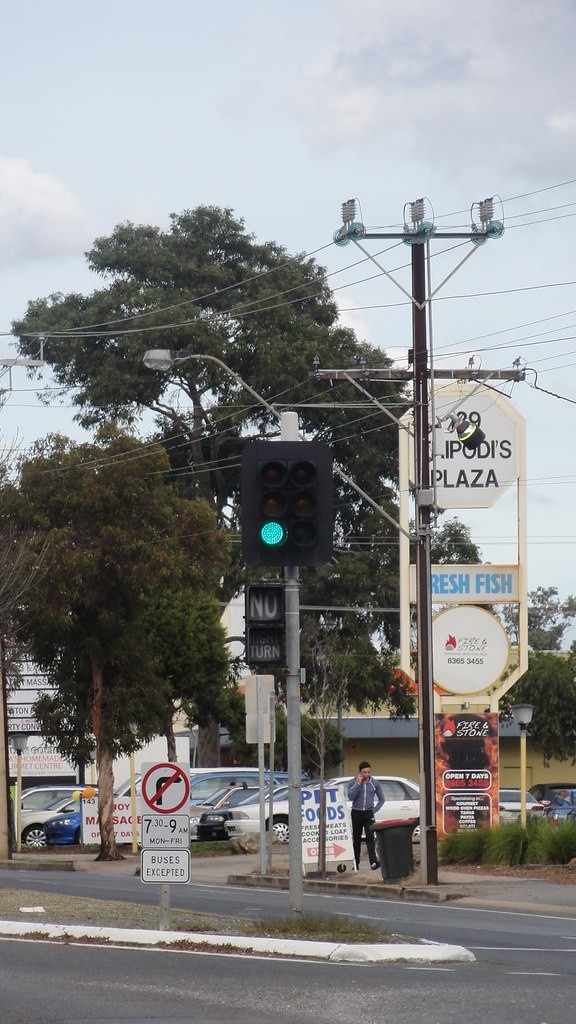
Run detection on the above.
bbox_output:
[370,816,419,884]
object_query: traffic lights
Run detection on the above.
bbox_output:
[239,441,333,567]
[245,583,285,666]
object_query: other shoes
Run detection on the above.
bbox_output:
[353,865,359,870]
[371,861,380,870]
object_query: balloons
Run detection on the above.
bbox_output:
[83,788,95,798]
[72,791,81,801]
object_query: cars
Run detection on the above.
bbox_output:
[190,781,327,840]
[528,783,576,807]
[223,775,421,844]
[189,771,310,807]
[542,790,576,825]
[499,790,545,821]
[43,811,82,844]
[20,791,114,848]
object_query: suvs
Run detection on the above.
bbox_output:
[21,784,99,813]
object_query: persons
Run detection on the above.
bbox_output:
[347,761,385,870]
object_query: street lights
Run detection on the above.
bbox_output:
[509,703,537,830]
[10,734,28,852]
[144,350,438,889]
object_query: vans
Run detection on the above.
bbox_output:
[113,767,272,798]
